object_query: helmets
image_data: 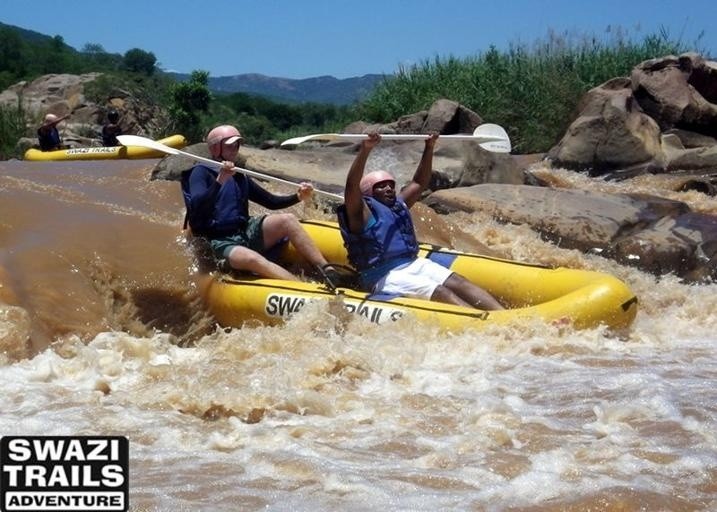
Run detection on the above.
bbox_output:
[208,125,245,158]
[45,113,57,122]
[359,171,396,196]
[108,110,119,121]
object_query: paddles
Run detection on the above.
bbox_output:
[116,135,345,204]
[14,103,88,138]
[281,123,511,154]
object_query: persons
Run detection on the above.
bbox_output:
[101,109,136,147]
[178,124,359,288]
[336,129,503,310]
[37,114,75,150]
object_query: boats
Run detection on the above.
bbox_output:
[194,218,638,336]
[24,134,186,161]
